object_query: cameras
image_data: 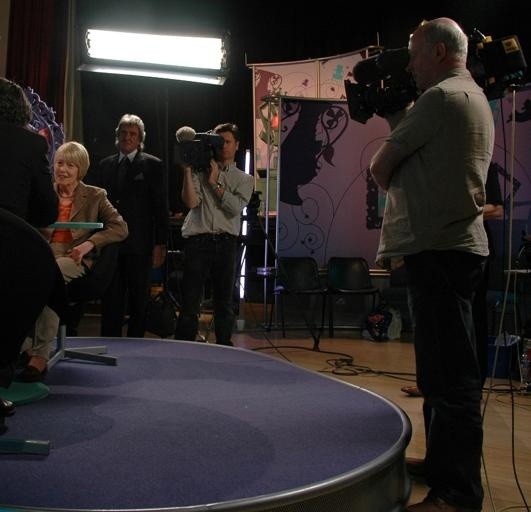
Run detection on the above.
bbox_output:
[343,29,526,125]
[175,126,224,171]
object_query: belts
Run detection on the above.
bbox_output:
[190,233,236,241]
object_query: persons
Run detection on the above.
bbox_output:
[370,17,495,512]
[0,76,58,232]
[12,142,129,383]
[174,123,256,347]
[401,160,506,396]
[94,114,171,339]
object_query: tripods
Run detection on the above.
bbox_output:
[206,216,322,349]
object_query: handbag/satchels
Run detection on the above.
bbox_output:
[366,304,393,339]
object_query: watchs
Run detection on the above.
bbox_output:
[211,182,223,191]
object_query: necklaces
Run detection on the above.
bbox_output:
[58,184,77,198]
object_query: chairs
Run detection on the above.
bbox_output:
[327,257,383,343]
[266,256,335,341]
[379,267,413,333]
[45,221,117,372]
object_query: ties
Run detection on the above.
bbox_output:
[116,157,127,203]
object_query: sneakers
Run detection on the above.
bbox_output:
[15,352,48,383]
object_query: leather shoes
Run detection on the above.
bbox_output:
[405,491,481,512]
[402,386,422,397]
[406,457,427,477]
[0,398,16,417]
